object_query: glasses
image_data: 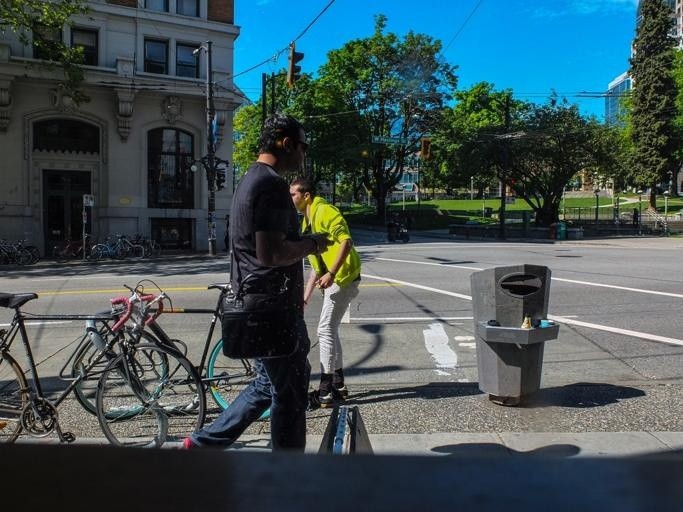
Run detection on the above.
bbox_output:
[286,135,309,151]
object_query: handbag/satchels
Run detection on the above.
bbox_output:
[219,294,298,358]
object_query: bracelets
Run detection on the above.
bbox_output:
[327,270,335,278]
[306,232,322,255]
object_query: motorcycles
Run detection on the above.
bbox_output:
[386,226,411,244]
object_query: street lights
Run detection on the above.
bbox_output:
[190,37,230,257]
[663,190,670,234]
[635,189,644,235]
[592,188,600,223]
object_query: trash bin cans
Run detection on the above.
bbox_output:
[470,264,559,407]
[549,222,567,240]
[484,207,493,218]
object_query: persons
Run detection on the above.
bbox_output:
[172,114,335,454]
[288,175,361,408]
[631,207,638,228]
[612,202,618,221]
[389,211,403,244]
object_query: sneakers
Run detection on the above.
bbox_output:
[317,385,348,407]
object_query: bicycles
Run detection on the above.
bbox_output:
[0,231,161,268]
[66,283,275,422]
[1,287,207,450]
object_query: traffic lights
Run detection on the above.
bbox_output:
[419,137,431,159]
[286,48,305,86]
